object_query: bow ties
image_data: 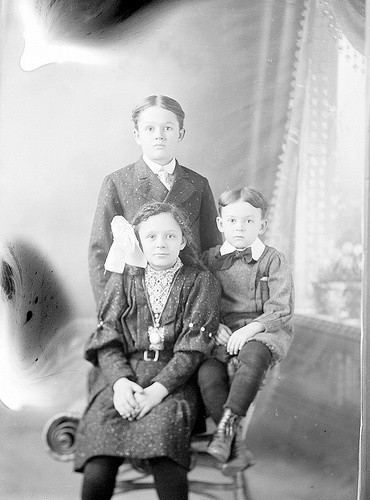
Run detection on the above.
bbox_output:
[222,249,252,269]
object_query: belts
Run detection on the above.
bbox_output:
[127,349,169,361]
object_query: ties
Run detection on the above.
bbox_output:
[157,170,171,190]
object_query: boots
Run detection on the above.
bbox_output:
[208,413,254,474]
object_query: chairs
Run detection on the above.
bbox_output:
[113,356,252,500]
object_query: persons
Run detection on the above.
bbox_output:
[73,203,221,500]
[200,186,293,475]
[88,95,222,312]
